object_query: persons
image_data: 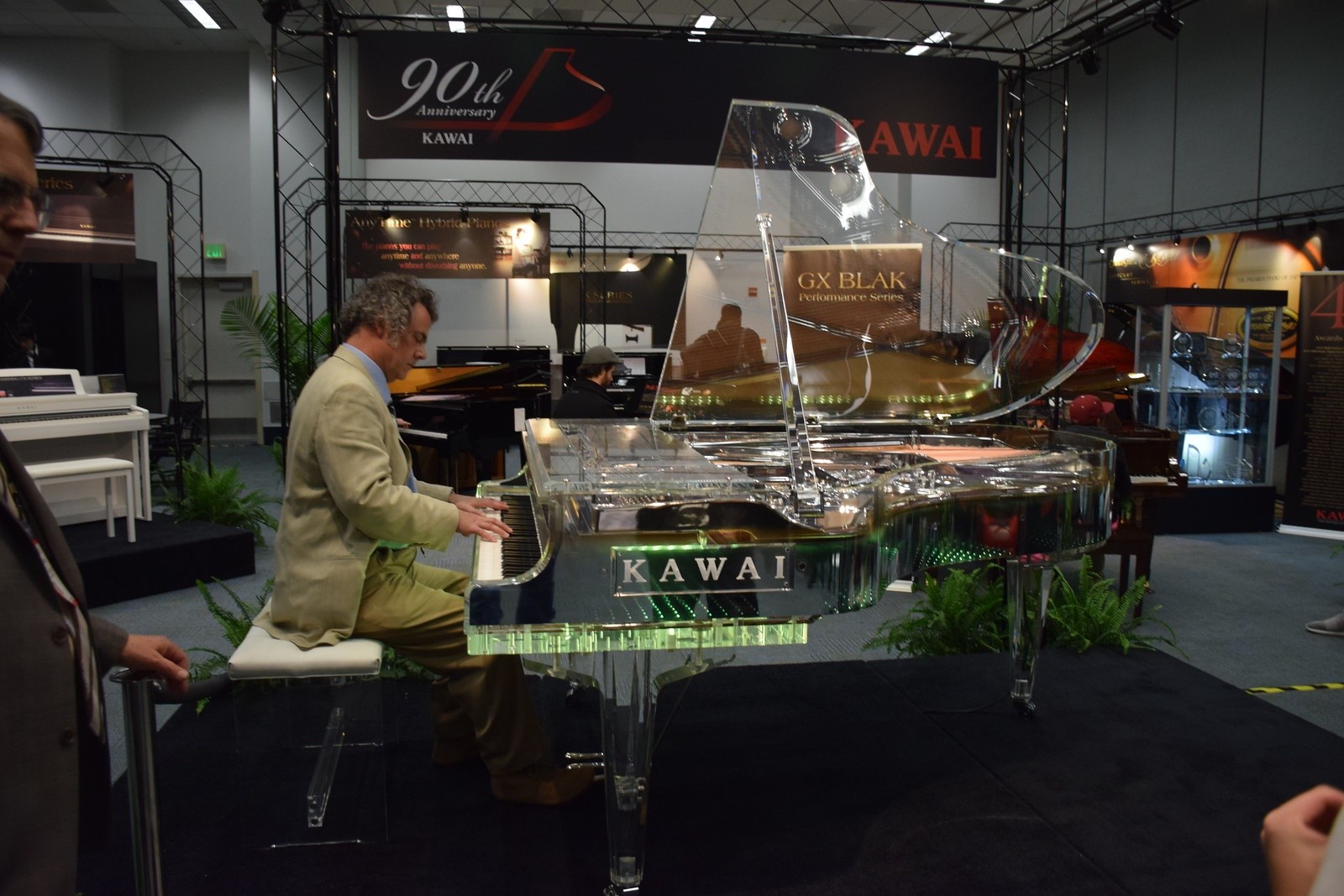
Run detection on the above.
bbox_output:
[496,231,506,255]
[1050,396,1130,531]
[552,346,623,418]
[0,323,53,397]
[1260,785,1344,895]
[685,306,769,383]
[1306,612,1344,636]
[251,274,595,804]
[939,464,1020,548]
[515,228,537,265]
[0,97,188,896]
[630,401,761,623]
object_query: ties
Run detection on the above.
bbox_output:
[1,466,106,741]
[386,398,396,418]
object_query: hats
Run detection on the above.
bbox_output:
[583,346,623,364]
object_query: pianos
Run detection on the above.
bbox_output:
[460,94,1123,896]
[986,290,1189,626]
[0,368,153,524]
[390,359,553,494]
[560,349,672,412]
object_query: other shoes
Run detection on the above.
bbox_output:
[1304,613,1344,637]
[492,761,595,805]
